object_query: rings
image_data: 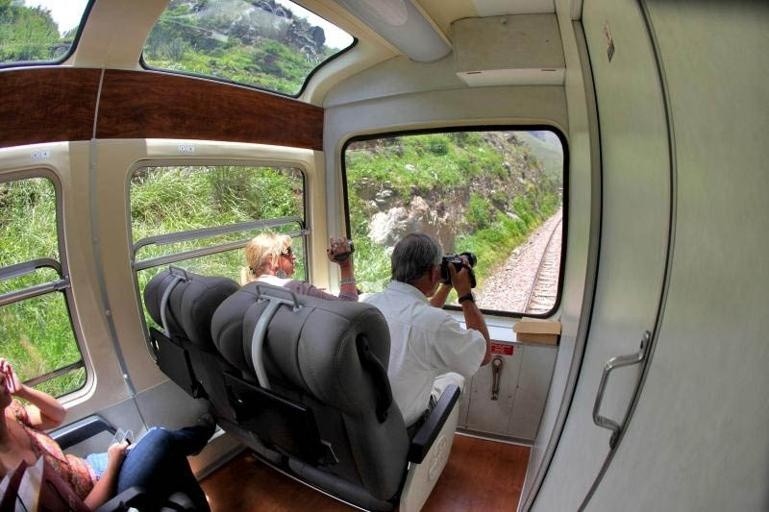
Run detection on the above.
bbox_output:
[335,242,338,248]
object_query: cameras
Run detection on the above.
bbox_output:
[326,240,355,255]
[440,251,477,287]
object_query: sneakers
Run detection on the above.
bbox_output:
[190,410,217,457]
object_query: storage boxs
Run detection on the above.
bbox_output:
[514,314,561,345]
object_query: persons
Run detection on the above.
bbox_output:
[242,227,360,304]
[357,232,493,442]
[0,356,216,511]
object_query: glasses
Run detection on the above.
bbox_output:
[281,247,292,256]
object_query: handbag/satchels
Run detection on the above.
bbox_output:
[1,454,94,511]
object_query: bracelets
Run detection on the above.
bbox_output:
[458,291,476,304]
[338,276,357,286]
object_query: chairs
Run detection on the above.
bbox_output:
[48,414,208,512]
[143,265,465,511]
